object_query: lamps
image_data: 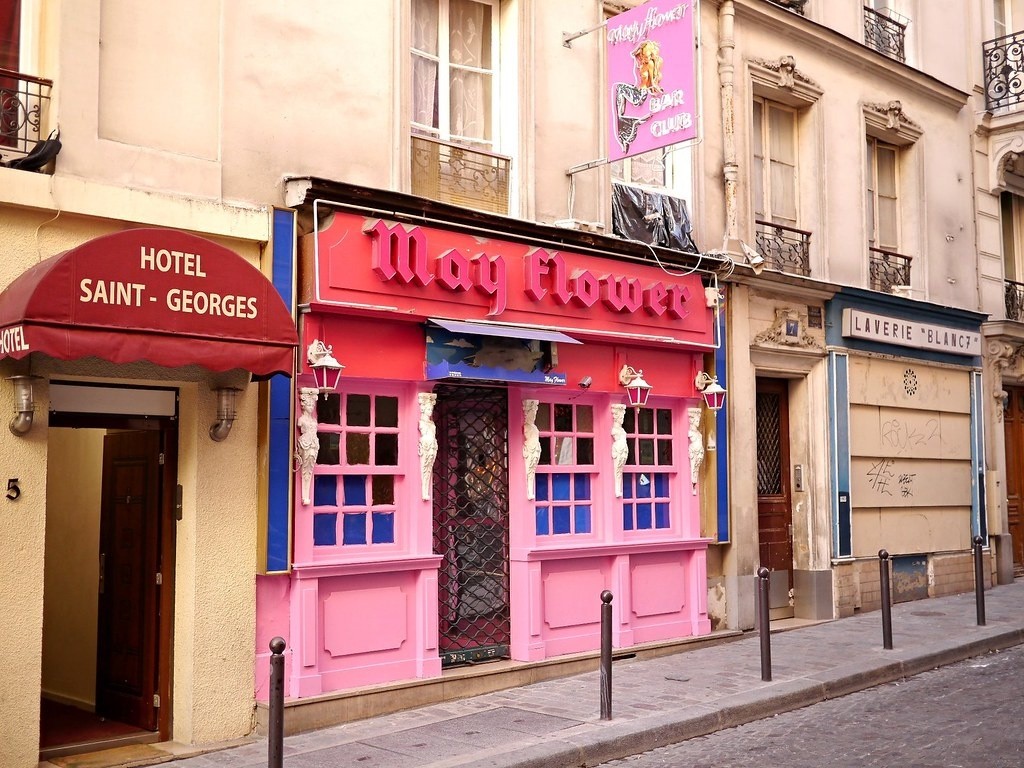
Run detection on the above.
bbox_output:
[618,364,653,415]
[695,370,727,417]
[307,338,346,401]
